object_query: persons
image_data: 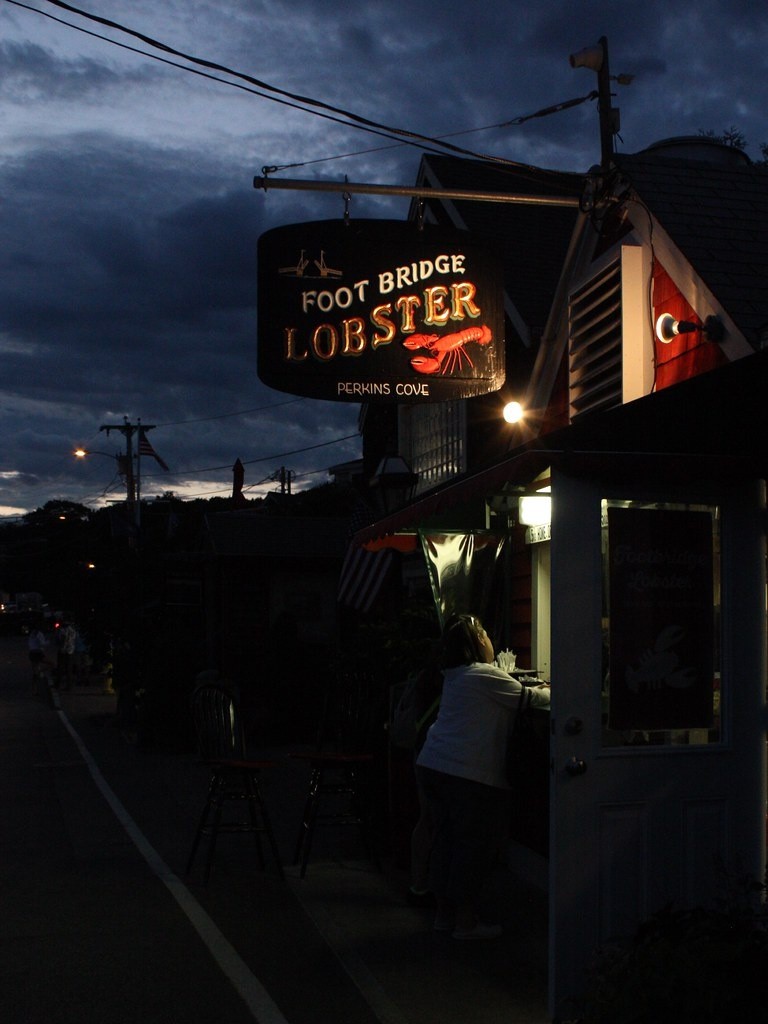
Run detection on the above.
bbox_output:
[27,620,50,696]
[409,612,551,938]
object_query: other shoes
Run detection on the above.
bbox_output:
[452,921,502,940]
[434,918,456,930]
[406,886,438,909]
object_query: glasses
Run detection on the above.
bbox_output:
[461,615,474,627]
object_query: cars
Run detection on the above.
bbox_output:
[0,600,42,636]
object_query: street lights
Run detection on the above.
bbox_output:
[74,449,136,554]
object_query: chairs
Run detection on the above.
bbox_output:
[291,688,387,869]
[185,684,288,882]
